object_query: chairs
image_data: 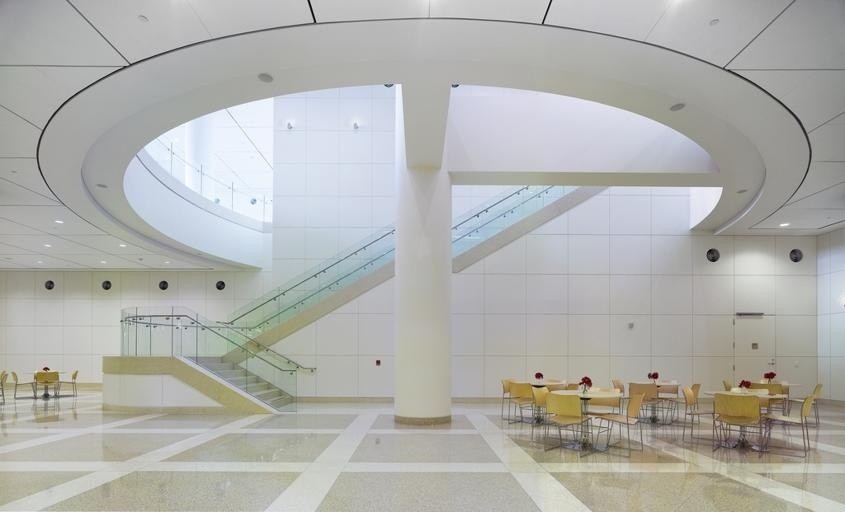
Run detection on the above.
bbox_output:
[0,366,79,405]
[503,373,825,458]
